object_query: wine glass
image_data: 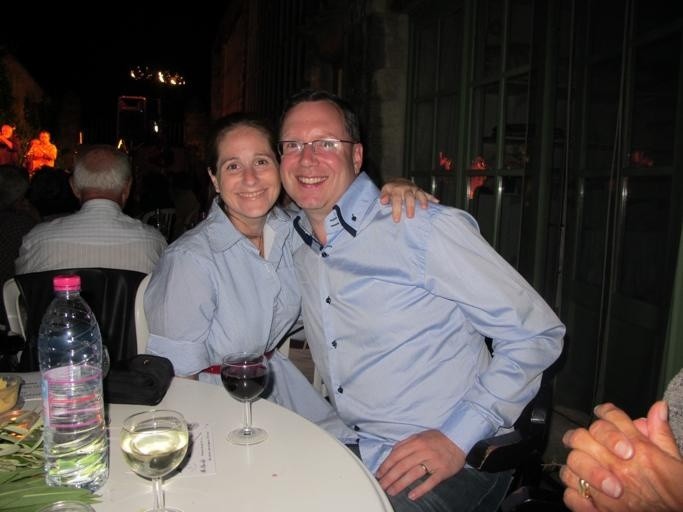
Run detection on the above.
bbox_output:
[120,408,189,512]
[220,351,270,447]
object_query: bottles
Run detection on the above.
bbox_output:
[37,275,111,497]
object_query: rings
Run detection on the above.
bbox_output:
[418,462,430,476]
[578,478,591,501]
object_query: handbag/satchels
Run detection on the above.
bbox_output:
[103,354,173,406]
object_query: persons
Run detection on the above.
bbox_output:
[23,130,57,182]
[14,144,168,275]
[1,123,21,165]
[143,108,440,447]
[1,162,42,326]
[269,81,569,511]
[27,150,81,217]
[558,369,683,512]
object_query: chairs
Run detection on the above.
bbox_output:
[465,362,568,510]
[3,206,175,366]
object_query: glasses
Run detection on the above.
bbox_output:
[276,138,355,158]
